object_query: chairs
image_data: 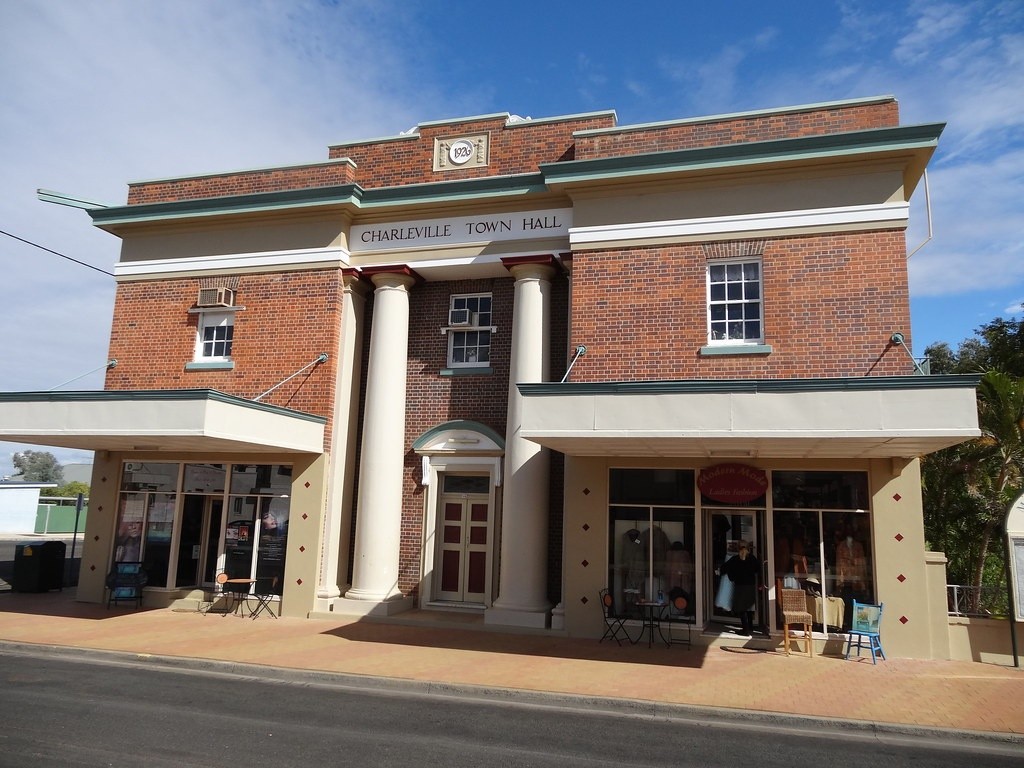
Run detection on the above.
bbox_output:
[204,573,231,617]
[599,588,634,646]
[248,577,279,620]
[846,599,886,665]
[781,589,813,658]
[666,586,693,650]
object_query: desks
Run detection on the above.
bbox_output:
[221,579,260,618]
[629,602,671,647]
[806,595,845,632]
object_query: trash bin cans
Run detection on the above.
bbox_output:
[12,542,68,592]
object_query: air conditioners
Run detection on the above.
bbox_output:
[197,287,233,307]
[448,309,473,327]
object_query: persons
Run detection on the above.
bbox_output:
[260,509,277,529]
[714,539,764,636]
[113,512,143,563]
[836,536,867,633]
